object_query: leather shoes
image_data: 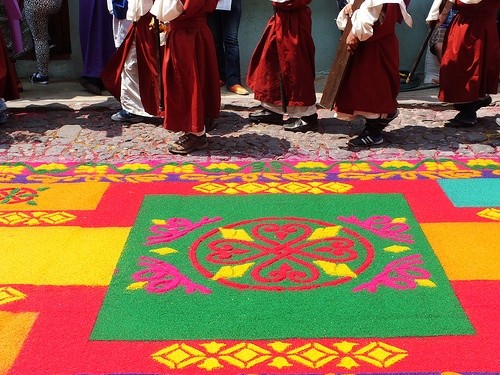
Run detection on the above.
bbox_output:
[249,108,283,124]
[283,113,319,131]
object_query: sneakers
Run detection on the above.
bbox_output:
[228,83,249,95]
[168,133,208,156]
[347,133,384,147]
[378,109,400,130]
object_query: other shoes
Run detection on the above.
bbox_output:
[80,77,103,94]
[111,109,144,123]
[445,116,478,128]
[28,71,50,84]
[454,96,491,110]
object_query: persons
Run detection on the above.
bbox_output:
[207,0,248,95]
[101,0,159,121]
[1,0,25,110]
[79,0,115,95]
[150,0,221,154]
[437,0,500,126]
[321,0,410,148]
[248,0,319,132]
[15,0,63,84]
[425,0,463,85]
